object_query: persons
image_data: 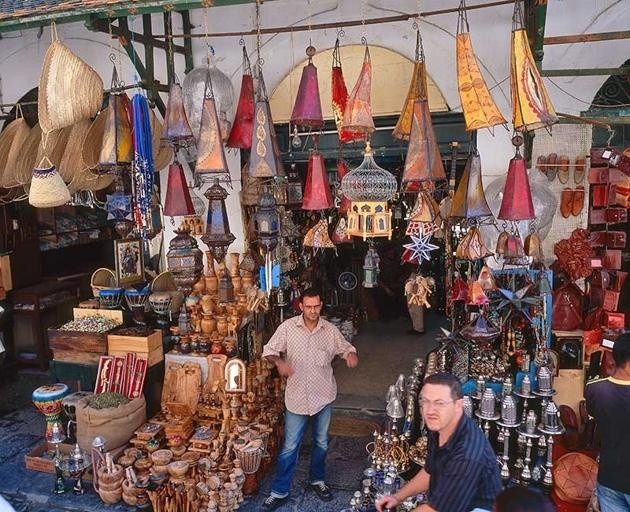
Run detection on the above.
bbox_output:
[259,288,361,511]
[584,329,630,512]
[403,268,427,335]
[373,370,504,512]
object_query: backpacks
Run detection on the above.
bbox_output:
[551,285,584,331]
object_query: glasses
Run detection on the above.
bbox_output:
[419,399,454,407]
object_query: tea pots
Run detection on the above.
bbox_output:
[365,430,412,472]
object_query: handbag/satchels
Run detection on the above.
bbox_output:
[0,108,173,208]
[37,41,104,134]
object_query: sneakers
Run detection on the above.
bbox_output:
[259,495,289,512]
[310,480,332,502]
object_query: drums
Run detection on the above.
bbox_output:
[149,292,171,329]
[32,383,69,445]
[61,391,93,444]
[232,437,263,499]
[79,300,98,308]
[99,288,123,310]
[243,424,277,480]
[125,286,152,332]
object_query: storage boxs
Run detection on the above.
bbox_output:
[48,309,124,367]
[108,331,162,370]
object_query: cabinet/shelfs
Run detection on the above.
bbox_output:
[11,280,75,369]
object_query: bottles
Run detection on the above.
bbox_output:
[178,336,236,357]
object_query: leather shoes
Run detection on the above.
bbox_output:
[558,400,593,451]
[536,153,586,218]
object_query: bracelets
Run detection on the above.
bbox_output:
[392,494,401,504]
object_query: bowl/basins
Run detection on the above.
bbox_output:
[98,446,189,507]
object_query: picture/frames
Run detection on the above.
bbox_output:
[114,239,146,286]
